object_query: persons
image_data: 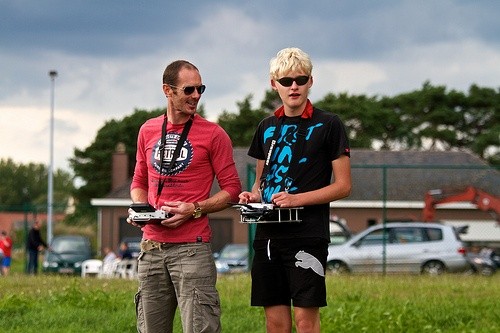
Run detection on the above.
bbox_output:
[104,242,132,278]
[0,232,12,274]
[127,60,242,332]
[28,221,50,275]
[239,48,351,333]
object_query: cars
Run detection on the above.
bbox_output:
[118,235,143,260]
[466,248,500,275]
[213,243,250,274]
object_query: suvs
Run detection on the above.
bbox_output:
[41,236,97,275]
[325,220,468,277]
[329,216,353,244]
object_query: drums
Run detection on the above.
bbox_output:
[241,202,304,223]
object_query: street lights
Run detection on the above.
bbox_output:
[46,68,58,246]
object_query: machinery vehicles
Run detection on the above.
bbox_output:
[408,184,500,228]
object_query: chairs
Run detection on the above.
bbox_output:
[112,260,137,279]
[81,259,103,278]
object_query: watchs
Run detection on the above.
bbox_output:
[192,202,201,218]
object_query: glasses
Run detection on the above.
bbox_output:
[166,83,205,97]
[276,75,311,87]
